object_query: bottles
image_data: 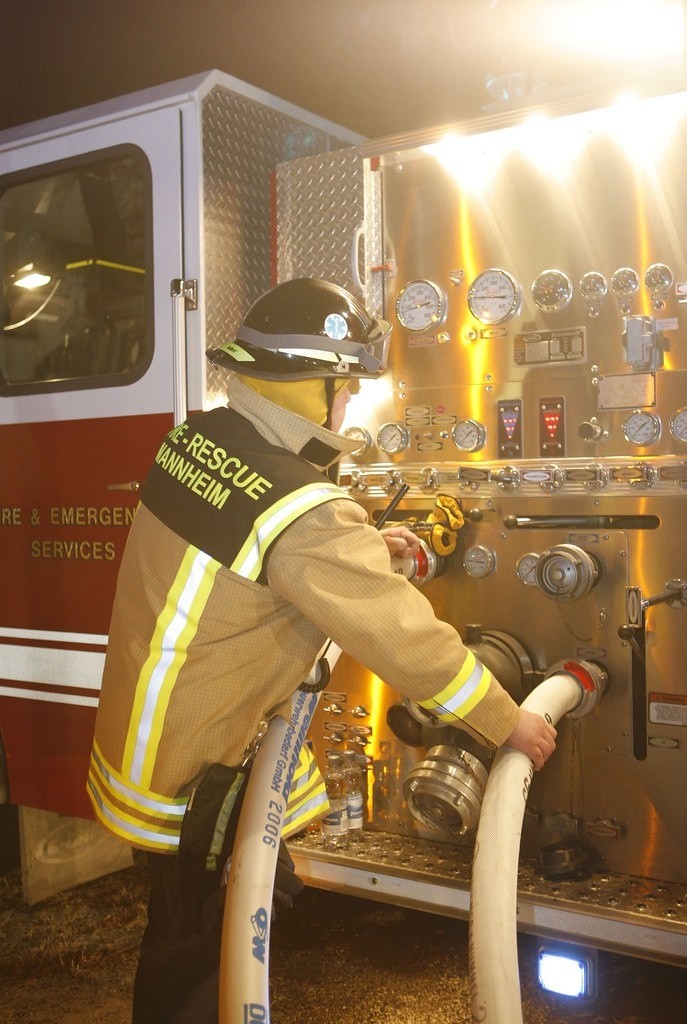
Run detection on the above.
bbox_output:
[322,755,348,851]
[340,751,364,842]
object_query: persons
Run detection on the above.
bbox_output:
[83,274,557,1024]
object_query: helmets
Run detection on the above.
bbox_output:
[204,277,394,383]
